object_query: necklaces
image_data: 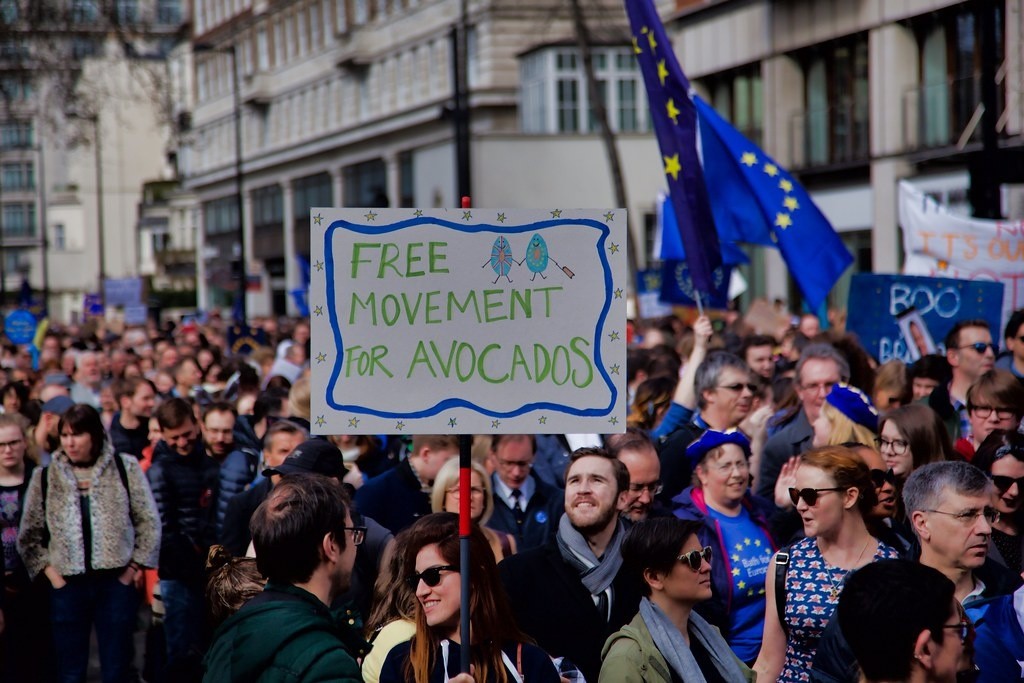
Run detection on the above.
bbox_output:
[816,536,873,597]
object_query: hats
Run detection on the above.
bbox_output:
[263,438,346,479]
[826,382,881,433]
[685,426,749,463]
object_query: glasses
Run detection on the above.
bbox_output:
[715,383,757,393]
[920,508,999,526]
[930,621,968,638]
[332,525,368,544]
[675,545,713,571]
[969,404,1017,421]
[407,565,458,589]
[787,486,863,507]
[874,436,909,454]
[1,440,23,450]
[991,475,1024,492]
[957,343,999,355]
[867,468,898,487]
[620,481,665,496]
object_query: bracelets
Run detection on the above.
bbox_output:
[129,565,138,572]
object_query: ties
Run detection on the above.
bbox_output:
[512,489,523,529]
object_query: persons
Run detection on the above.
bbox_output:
[498,309,1024,682]
[907,321,927,356]
[0,312,561,683]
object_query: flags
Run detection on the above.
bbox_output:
[625,0,853,329]
